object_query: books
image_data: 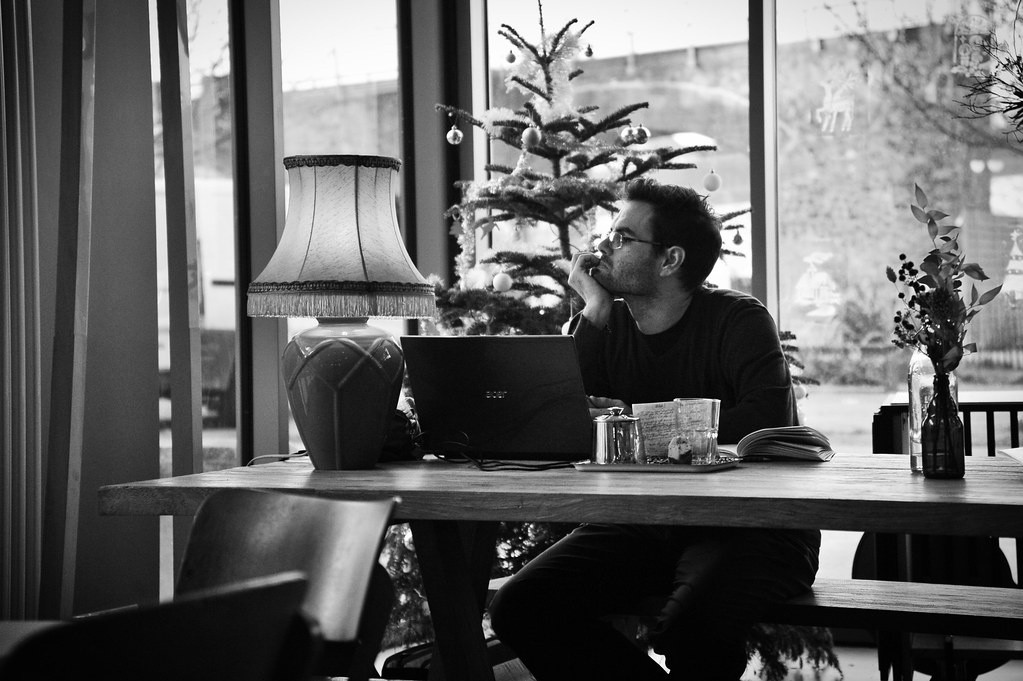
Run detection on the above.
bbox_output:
[717,424,837,464]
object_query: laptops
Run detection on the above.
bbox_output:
[400,334,594,460]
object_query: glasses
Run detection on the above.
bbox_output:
[608,230,666,251]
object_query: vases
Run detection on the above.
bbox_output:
[919,374,969,482]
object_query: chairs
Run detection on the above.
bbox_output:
[159,485,400,680]
[865,401,1023,680]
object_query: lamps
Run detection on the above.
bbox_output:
[245,151,436,472]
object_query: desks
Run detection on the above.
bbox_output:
[92,445,1022,540]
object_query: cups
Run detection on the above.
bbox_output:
[592,406,640,465]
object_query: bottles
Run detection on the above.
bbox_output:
[673,398,721,465]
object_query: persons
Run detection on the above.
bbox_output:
[482,175,820,680]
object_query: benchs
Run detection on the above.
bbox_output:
[478,556,1023,680]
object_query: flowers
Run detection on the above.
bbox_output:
[883,181,1006,376]
